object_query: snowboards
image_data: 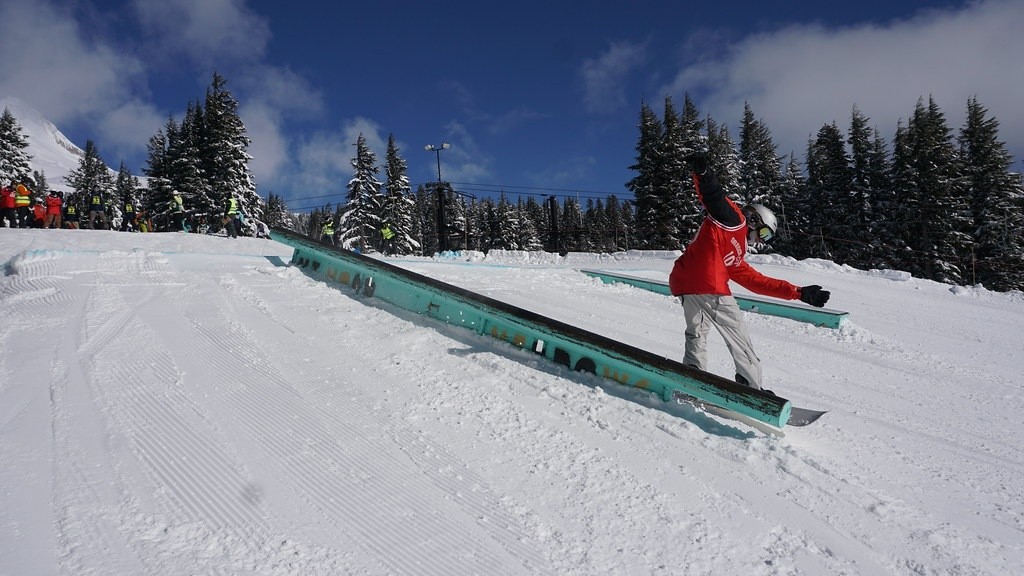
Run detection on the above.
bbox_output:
[785,406,828,427]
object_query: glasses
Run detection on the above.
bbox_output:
[750,214,775,243]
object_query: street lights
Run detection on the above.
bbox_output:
[424,142,450,251]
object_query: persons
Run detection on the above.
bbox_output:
[321,213,335,246]
[89,185,109,230]
[380,223,399,257]
[223,192,238,239]
[669,151,831,396]
[120,197,136,231]
[0,179,79,229]
[165,190,185,233]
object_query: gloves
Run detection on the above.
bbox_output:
[683,151,707,175]
[799,285,830,308]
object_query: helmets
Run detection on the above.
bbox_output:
[742,204,777,247]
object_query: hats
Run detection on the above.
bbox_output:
[21,177,31,182]
[11,180,18,186]
